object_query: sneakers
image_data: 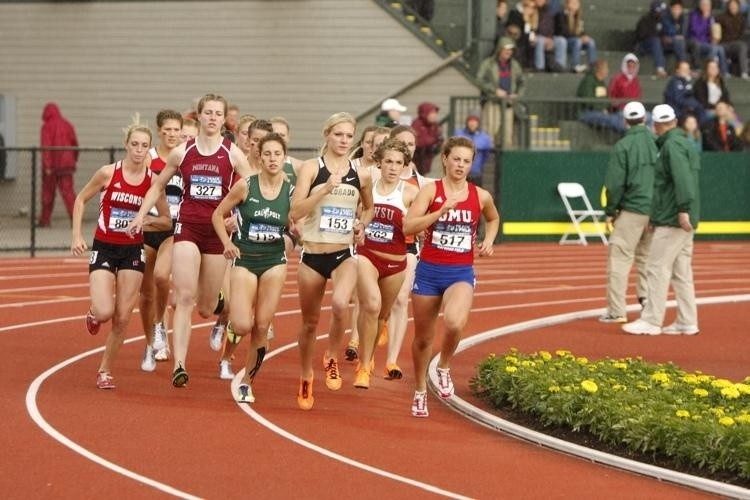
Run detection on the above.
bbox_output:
[344,340,359,361]
[227,321,242,345]
[436,363,454,399]
[574,64,587,73]
[742,73,749,81]
[622,318,661,336]
[151,321,171,361]
[411,390,429,418]
[322,351,342,391]
[639,300,648,316]
[214,289,224,315]
[599,315,627,322]
[267,322,273,341]
[96,369,116,389]
[298,370,314,410]
[722,72,732,80]
[383,364,402,380]
[354,360,375,389]
[218,359,235,379]
[86,306,101,335]
[172,360,189,387]
[141,345,157,373]
[238,384,255,403]
[661,321,699,335]
[210,318,227,352]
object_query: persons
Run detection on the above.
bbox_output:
[402,135,500,417]
[715,0,750,81]
[563,0,598,73]
[383,125,436,380]
[681,115,703,154]
[266,119,305,343]
[597,100,658,322]
[494,0,521,53]
[453,114,492,240]
[509,1,536,69]
[621,104,701,336]
[688,0,734,81]
[138,109,184,371]
[345,124,381,360]
[632,0,669,77]
[607,54,654,131]
[209,118,273,380]
[533,0,570,73]
[71,112,173,388]
[694,60,750,152]
[126,93,253,386]
[575,57,629,132]
[662,57,719,152]
[353,134,430,388]
[374,98,408,130]
[211,132,301,404]
[476,37,529,150]
[221,105,240,144]
[289,111,376,411]
[36,102,79,228]
[661,0,692,61]
[370,125,392,161]
[410,101,445,179]
[180,117,201,144]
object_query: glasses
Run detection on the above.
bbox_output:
[628,64,636,68]
[523,3,536,10]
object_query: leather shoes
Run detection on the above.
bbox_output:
[655,70,667,79]
[690,71,699,77]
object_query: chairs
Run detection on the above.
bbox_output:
[557,182,611,246]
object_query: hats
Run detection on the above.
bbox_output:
[623,102,646,120]
[651,104,676,123]
[466,116,479,128]
[381,99,407,112]
